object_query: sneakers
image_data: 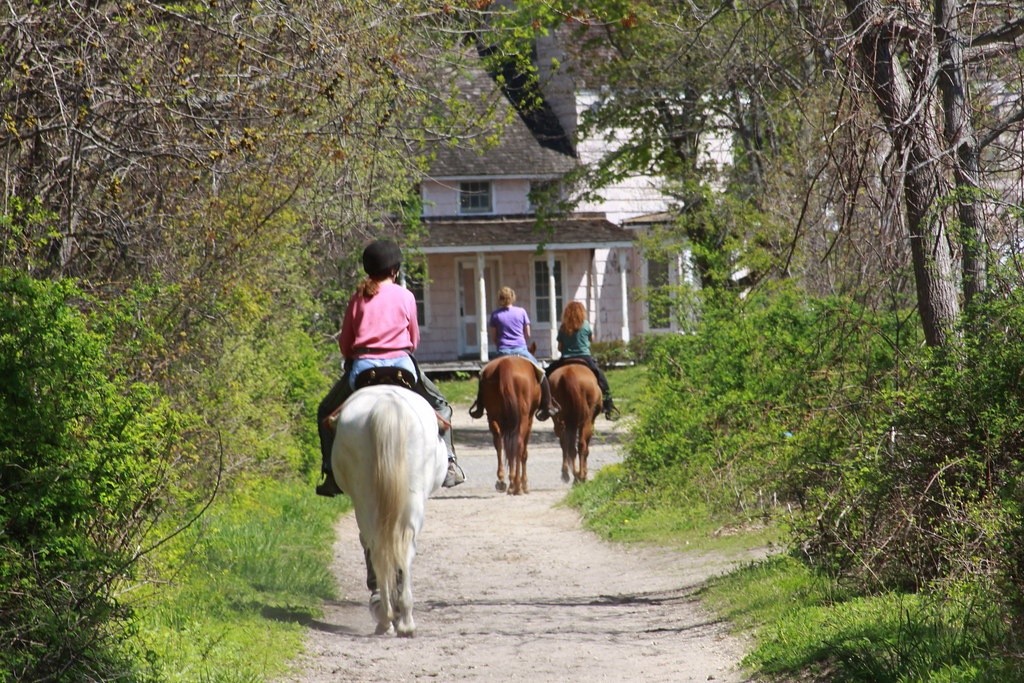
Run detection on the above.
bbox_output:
[445,467,464,487]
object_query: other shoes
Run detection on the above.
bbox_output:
[606,406,617,417]
[473,409,483,417]
[538,412,549,419]
[317,477,342,497]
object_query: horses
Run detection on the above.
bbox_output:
[481,354,542,495]
[548,362,603,488]
[330,383,449,638]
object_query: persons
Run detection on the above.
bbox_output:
[470,286,559,418]
[539,301,615,410]
[314,240,464,496]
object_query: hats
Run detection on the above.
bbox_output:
[361,241,403,277]
